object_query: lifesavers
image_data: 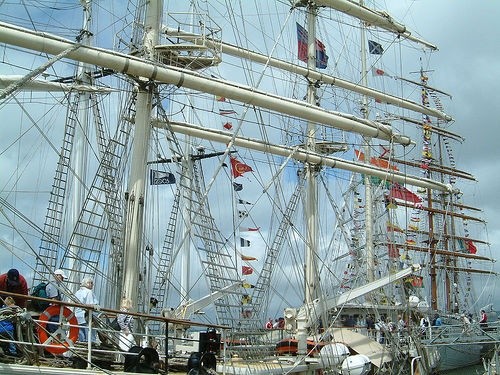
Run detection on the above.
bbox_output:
[37,304,79,353]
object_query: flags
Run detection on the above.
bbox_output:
[296,22,329,69]
[150,169,175,185]
[458,238,477,254]
[355,149,404,190]
[368,40,384,54]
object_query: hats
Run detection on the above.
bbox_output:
[7,269,20,286]
[54,269,68,279]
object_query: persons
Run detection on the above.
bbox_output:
[344,310,488,343]
[265,317,285,330]
[0,268,136,364]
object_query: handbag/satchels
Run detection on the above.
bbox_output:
[109,316,127,331]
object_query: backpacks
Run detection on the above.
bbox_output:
[30,281,61,313]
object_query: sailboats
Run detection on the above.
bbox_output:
[0,1,500,375]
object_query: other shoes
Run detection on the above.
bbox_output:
[45,355,55,358]
